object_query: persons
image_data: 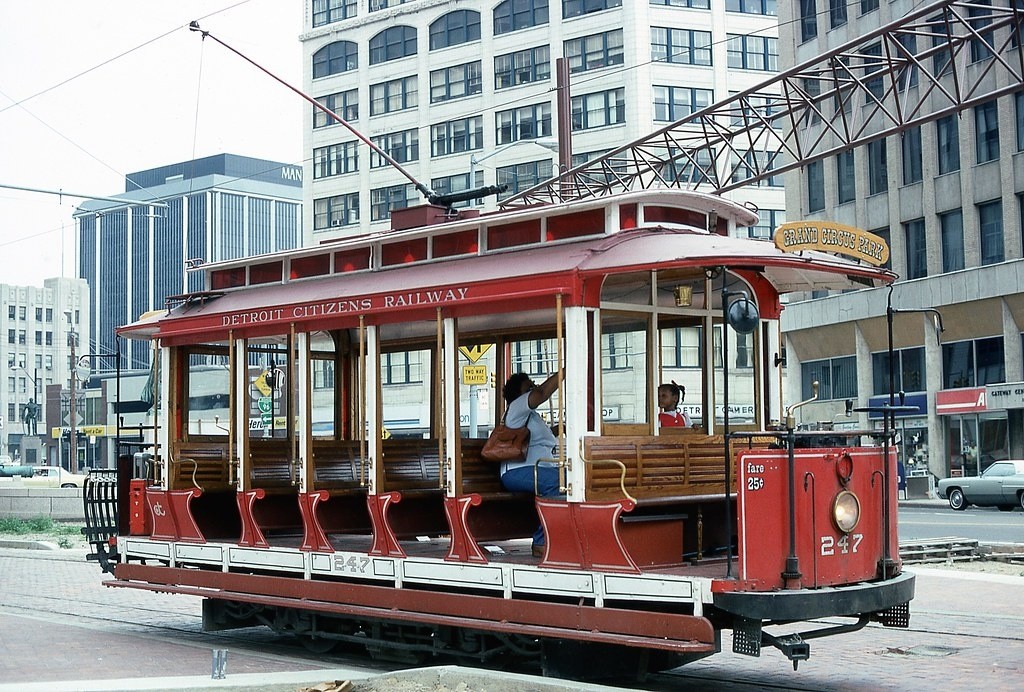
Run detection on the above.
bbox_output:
[500,369,565,556]
[967,441,981,457]
[23,398,38,436]
[658,380,700,430]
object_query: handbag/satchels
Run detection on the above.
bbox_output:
[480,407,531,462]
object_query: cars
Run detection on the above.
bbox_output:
[0,464,91,489]
[41,457,47,466]
[0,455,21,464]
[938,459,1024,511]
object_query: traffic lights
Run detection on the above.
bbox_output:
[491,372,496,389]
[62,433,71,443]
[542,412,547,424]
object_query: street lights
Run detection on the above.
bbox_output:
[266,353,278,437]
[468,139,561,441]
[10,366,37,435]
[63,309,78,474]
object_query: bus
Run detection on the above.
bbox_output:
[79,186,916,687]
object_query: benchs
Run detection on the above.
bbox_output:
[171,436,778,576]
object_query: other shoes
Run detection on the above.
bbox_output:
[531,544,545,557]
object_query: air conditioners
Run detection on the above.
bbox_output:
[332,220,342,227]
